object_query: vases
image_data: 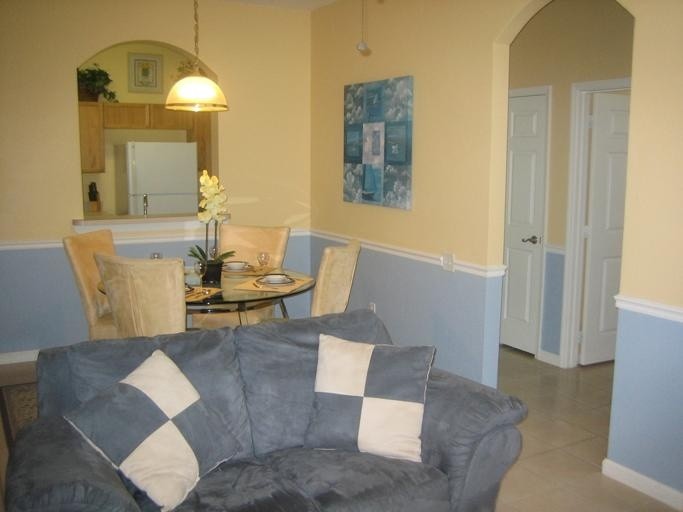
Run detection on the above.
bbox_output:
[199,263,222,285]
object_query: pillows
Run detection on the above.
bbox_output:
[61,351,243,511]
[306,333,437,472]
[237,309,396,456]
[67,328,255,497]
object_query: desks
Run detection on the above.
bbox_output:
[98,262,316,329]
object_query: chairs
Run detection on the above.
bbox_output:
[62,229,117,341]
[310,239,361,319]
[192,225,291,328]
[93,251,186,338]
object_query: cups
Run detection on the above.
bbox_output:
[150,252,162,259]
[194,261,208,296]
[257,252,270,272]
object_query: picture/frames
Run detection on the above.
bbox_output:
[128,52,164,94]
[342,76,413,211]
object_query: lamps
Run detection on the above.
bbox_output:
[164,0,228,111]
[357,0,368,52]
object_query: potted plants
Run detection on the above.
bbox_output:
[77,64,118,102]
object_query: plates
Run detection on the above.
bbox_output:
[186,281,195,293]
[223,261,251,273]
[256,274,295,287]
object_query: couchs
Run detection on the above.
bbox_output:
[2,307,530,512]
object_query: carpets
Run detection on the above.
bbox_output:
[1,382,40,453]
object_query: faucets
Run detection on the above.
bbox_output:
[141,193,149,216]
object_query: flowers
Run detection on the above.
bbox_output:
[189,170,237,261]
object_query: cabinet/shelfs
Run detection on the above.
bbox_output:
[105,104,193,131]
[186,112,211,176]
[79,104,103,173]
